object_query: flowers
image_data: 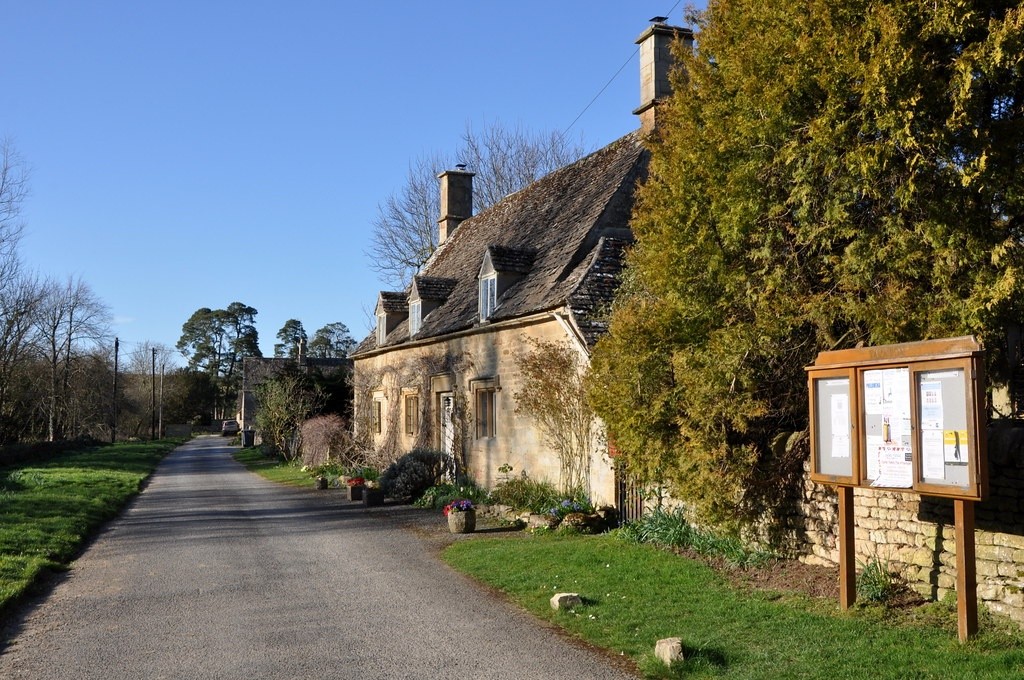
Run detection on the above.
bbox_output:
[347,475,384,491]
[302,464,330,480]
[442,500,472,517]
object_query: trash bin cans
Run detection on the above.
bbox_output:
[241,429,256,447]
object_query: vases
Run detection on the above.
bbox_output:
[346,486,364,501]
[448,509,477,535]
[315,477,329,490]
[362,490,385,508]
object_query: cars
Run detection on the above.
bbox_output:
[222,420,241,435]
